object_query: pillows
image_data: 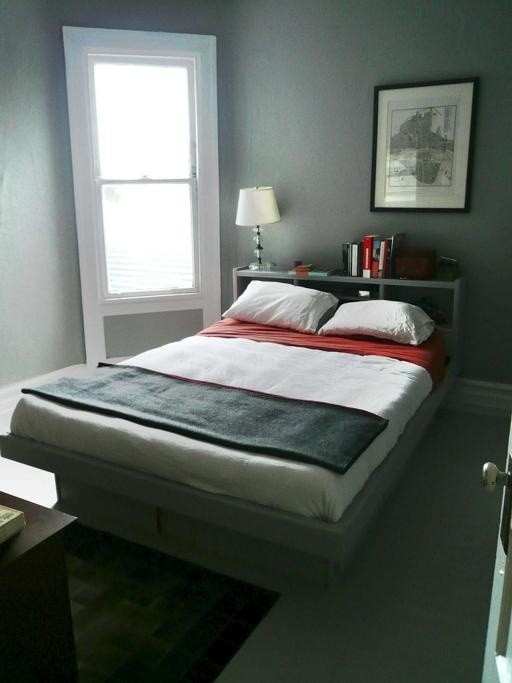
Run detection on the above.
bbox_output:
[221,280,339,333]
[315,298,437,346]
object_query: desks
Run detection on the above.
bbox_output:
[0,491,79,682]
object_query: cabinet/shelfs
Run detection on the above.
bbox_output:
[233,265,468,376]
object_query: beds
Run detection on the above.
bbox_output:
[0,317,455,586]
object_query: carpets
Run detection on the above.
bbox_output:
[64,519,279,682]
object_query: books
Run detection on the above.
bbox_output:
[287,266,339,276]
[341,231,406,279]
[0,503,27,545]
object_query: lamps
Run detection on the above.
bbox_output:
[234,185,282,272]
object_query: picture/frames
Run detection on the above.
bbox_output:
[369,75,478,213]
